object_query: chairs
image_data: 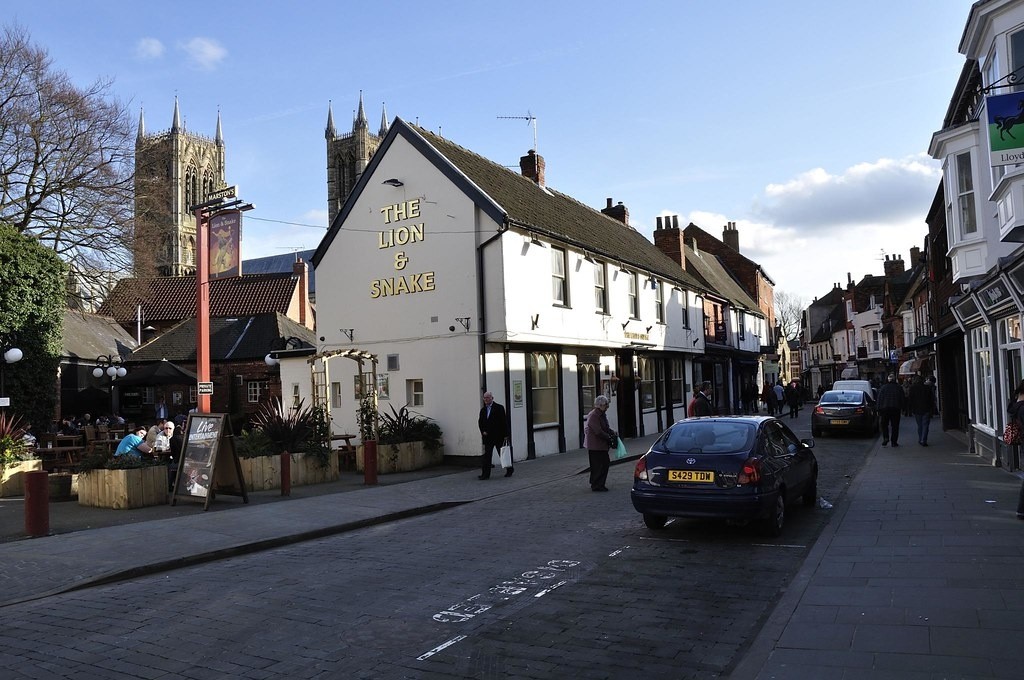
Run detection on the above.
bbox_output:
[38,422,135,473]
[677,430,748,451]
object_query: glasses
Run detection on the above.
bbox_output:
[165,428,172,429]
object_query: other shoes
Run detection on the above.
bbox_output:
[593,486,608,492]
[892,444,899,447]
[919,441,927,446]
[478,475,489,480]
[882,440,888,446]
[504,469,514,477]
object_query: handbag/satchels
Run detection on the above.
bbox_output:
[616,437,627,458]
[500,439,512,469]
[762,401,768,412]
[1002,421,1024,447]
[815,393,819,400]
[738,398,742,409]
[610,433,618,449]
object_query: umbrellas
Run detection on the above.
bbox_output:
[100,357,223,389]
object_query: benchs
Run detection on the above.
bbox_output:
[339,446,355,465]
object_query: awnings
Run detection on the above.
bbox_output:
[898,357,929,375]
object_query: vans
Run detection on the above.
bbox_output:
[833,379,874,402]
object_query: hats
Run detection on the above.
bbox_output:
[736,430,744,437]
[790,382,796,388]
[188,470,199,477]
[828,383,832,385]
[887,374,895,382]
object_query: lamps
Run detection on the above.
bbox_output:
[382,179,404,188]
[531,233,546,248]
[582,248,598,265]
[674,282,682,292]
[632,370,641,391]
[696,290,705,300]
[619,261,631,275]
[647,272,658,289]
[610,370,620,396]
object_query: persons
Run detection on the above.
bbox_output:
[762,381,808,418]
[113,419,184,464]
[911,376,936,447]
[155,396,168,419]
[478,392,514,479]
[1007,379,1024,519]
[689,381,712,417]
[57,414,79,436]
[19,420,32,438]
[900,379,913,417]
[817,382,833,397]
[95,416,108,424]
[750,383,759,413]
[583,395,615,492]
[114,411,125,424]
[79,414,90,426]
[876,374,906,447]
[175,411,187,421]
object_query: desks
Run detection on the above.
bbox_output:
[31,446,85,463]
[55,435,83,447]
[321,434,356,466]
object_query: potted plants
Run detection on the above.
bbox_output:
[229,396,339,492]
[0,411,42,498]
[76,443,171,510]
[356,403,445,475]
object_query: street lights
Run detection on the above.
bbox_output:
[92,353,126,417]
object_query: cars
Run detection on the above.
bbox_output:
[811,390,880,438]
[631,416,818,532]
[642,394,652,409]
